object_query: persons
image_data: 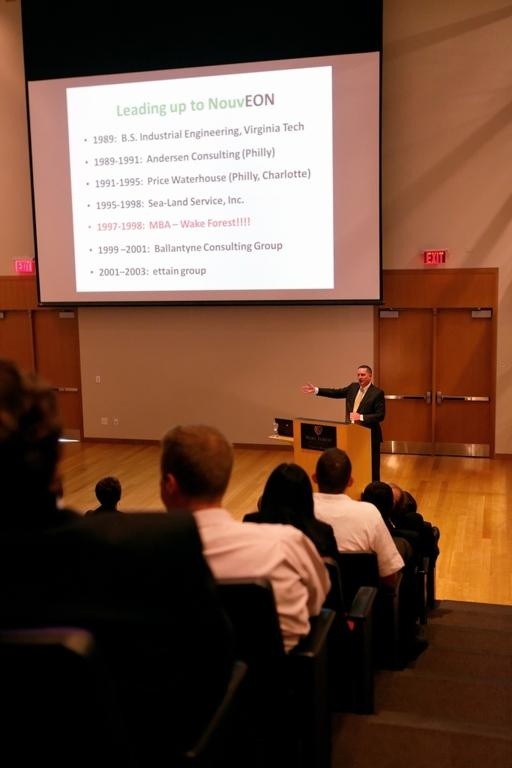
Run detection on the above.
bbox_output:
[157,422,332,654]
[234,462,339,557]
[300,364,386,484]
[312,446,406,590]
[0,357,223,768]
[82,476,122,516]
[359,480,443,613]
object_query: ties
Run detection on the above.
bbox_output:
[353,390,365,412]
